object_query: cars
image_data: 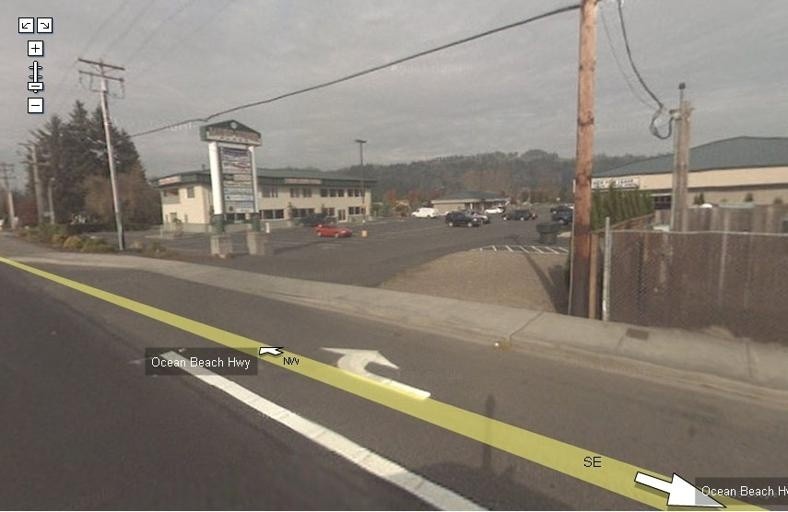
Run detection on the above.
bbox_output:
[484,206,507,214]
[550,206,573,226]
[502,209,538,221]
[299,213,337,227]
[313,224,352,239]
[445,211,488,228]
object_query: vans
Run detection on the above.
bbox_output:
[411,207,439,218]
